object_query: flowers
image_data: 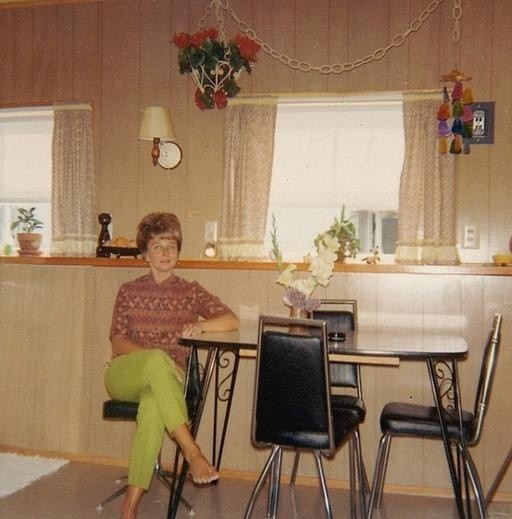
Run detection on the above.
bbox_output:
[170,26,261,110]
[269,212,343,313]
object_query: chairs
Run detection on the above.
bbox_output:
[367,314,502,518]
[244,316,366,518]
[97,348,202,515]
[290,300,369,496]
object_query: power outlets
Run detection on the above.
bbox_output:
[463,221,480,249]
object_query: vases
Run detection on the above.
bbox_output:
[289,308,309,334]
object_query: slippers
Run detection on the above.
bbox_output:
[188,452,219,488]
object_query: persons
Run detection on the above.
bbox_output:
[104,211,240,519]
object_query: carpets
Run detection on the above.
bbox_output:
[0,451,71,500]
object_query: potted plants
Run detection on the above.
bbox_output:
[314,204,362,262]
[9,206,46,254]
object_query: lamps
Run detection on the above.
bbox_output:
[136,106,183,170]
